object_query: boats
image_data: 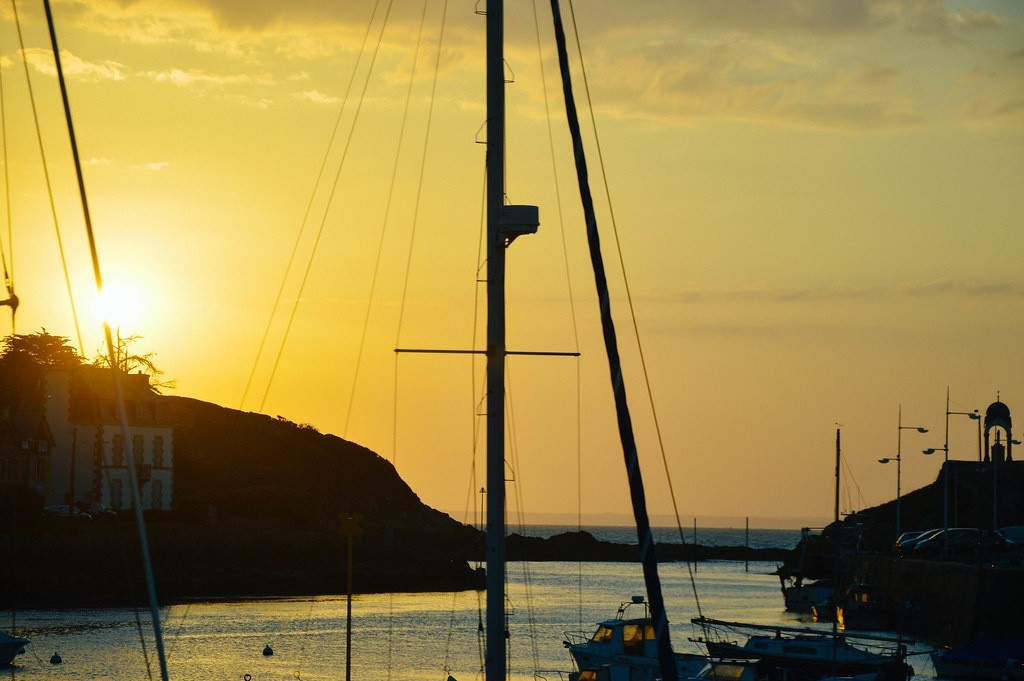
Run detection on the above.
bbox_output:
[0,630,32,668]
[561,594,1024,681]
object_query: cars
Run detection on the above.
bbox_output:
[895,526,1024,568]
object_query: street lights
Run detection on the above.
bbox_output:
[878,404,930,544]
[922,384,981,552]
